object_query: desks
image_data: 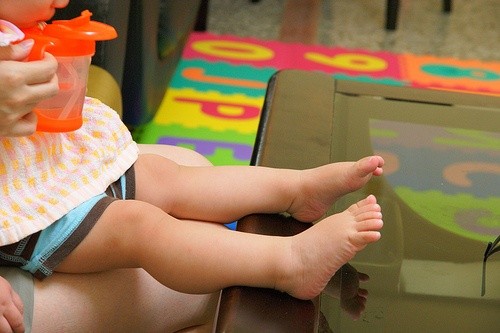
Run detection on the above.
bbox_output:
[206,67,500,333]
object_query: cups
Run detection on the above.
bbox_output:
[21,23,94,133]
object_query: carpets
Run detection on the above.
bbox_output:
[136,30,500,308]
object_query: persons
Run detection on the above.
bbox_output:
[1,36,230,333]
[1,0,384,333]
